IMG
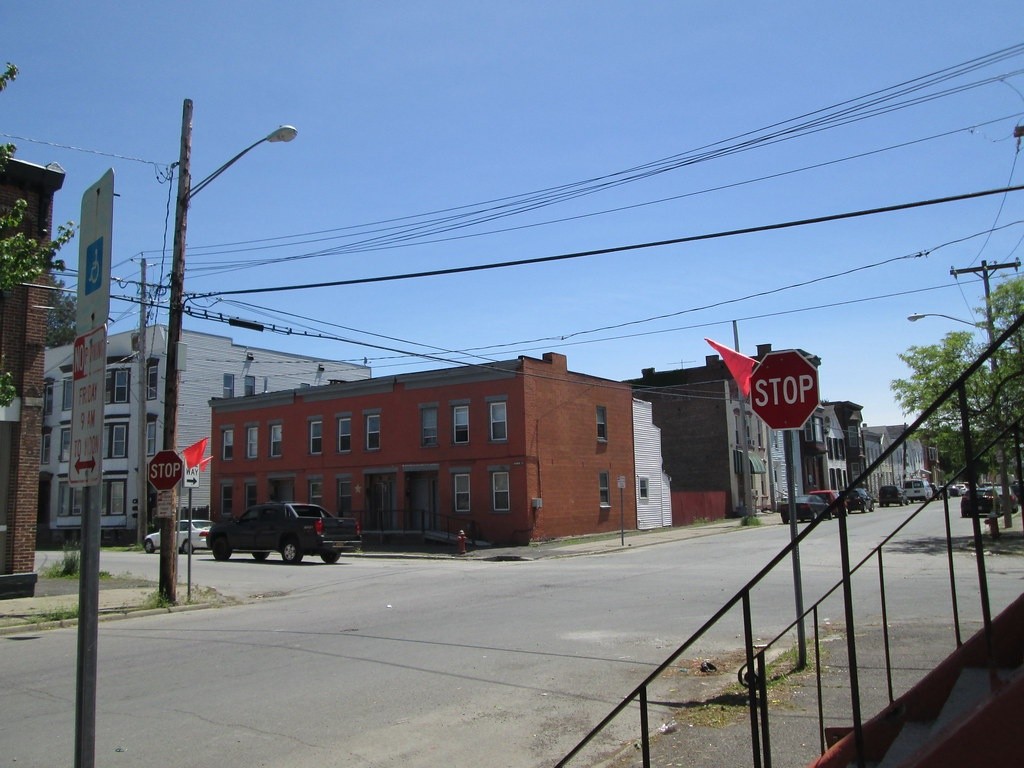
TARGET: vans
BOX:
[903,479,935,503]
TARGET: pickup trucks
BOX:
[204,501,362,566]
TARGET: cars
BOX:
[841,487,876,514]
[878,485,910,507]
[808,489,849,520]
[929,480,968,500]
[781,494,834,525]
[144,519,216,554]
[960,488,1001,519]
[984,486,1020,515]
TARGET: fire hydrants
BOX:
[458,528,469,554]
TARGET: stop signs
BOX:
[148,449,184,492]
[748,350,821,430]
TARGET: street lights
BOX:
[905,311,1015,530]
[157,120,301,608]
